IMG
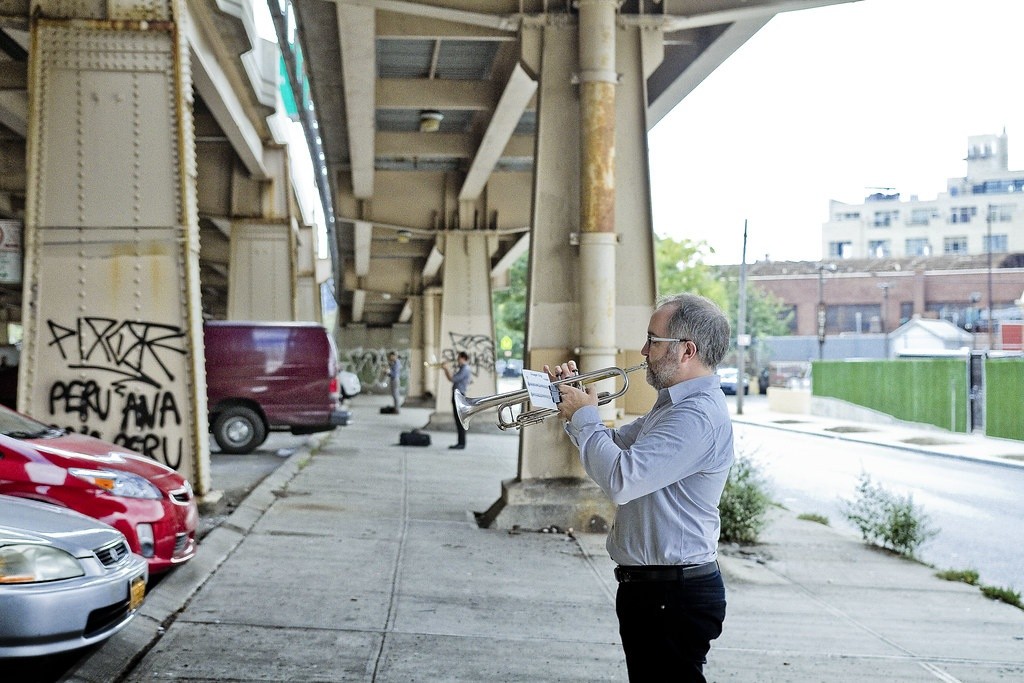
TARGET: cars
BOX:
[716,367,749,395]
[0,397,198,575]
[0,490,149,661]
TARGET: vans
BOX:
[758,361,807,395]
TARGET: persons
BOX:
[441,352,474,450]
[386,352,402,411]
[543,293,736,683]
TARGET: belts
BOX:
[614,560,719,582]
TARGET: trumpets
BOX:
[451,362,649,432]
[423,359,458,369]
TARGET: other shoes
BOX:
[449,444,464,449]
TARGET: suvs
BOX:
[1,321,361,454]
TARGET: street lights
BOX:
[816,263,838,360]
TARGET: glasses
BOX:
[647,335,692,348]
